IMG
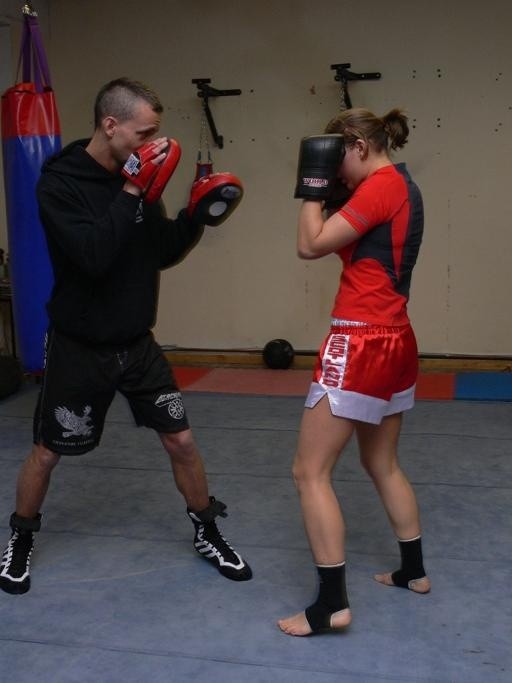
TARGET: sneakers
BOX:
[190,501,252,582]
[0,510,42,597]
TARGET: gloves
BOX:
[293,131,347,206]
[121,143,158,191]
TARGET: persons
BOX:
[269,104,436,641]
[1,73,255,597]
